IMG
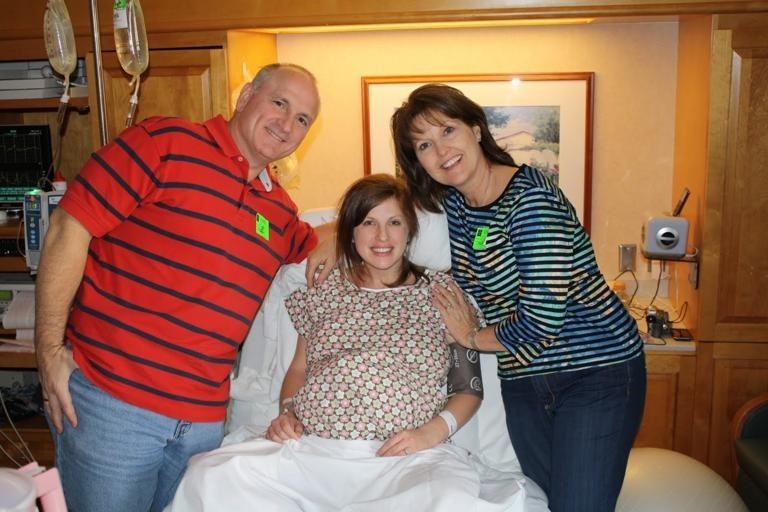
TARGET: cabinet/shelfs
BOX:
[0,30,276,469]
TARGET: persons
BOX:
[264,171,486,457]
[34,62,339,512]
[303,83,648,512]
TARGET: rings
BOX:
[43,398,48,401]
[447,302,454,309]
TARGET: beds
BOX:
[235,201,549,511]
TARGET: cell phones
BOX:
[672,327,695,341]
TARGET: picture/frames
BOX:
[359,71,593,240]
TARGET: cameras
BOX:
[645,305,668,338]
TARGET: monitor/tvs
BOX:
[0,123,54,216]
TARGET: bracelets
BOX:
[439,410,458,438]
[470,326,482,351]
